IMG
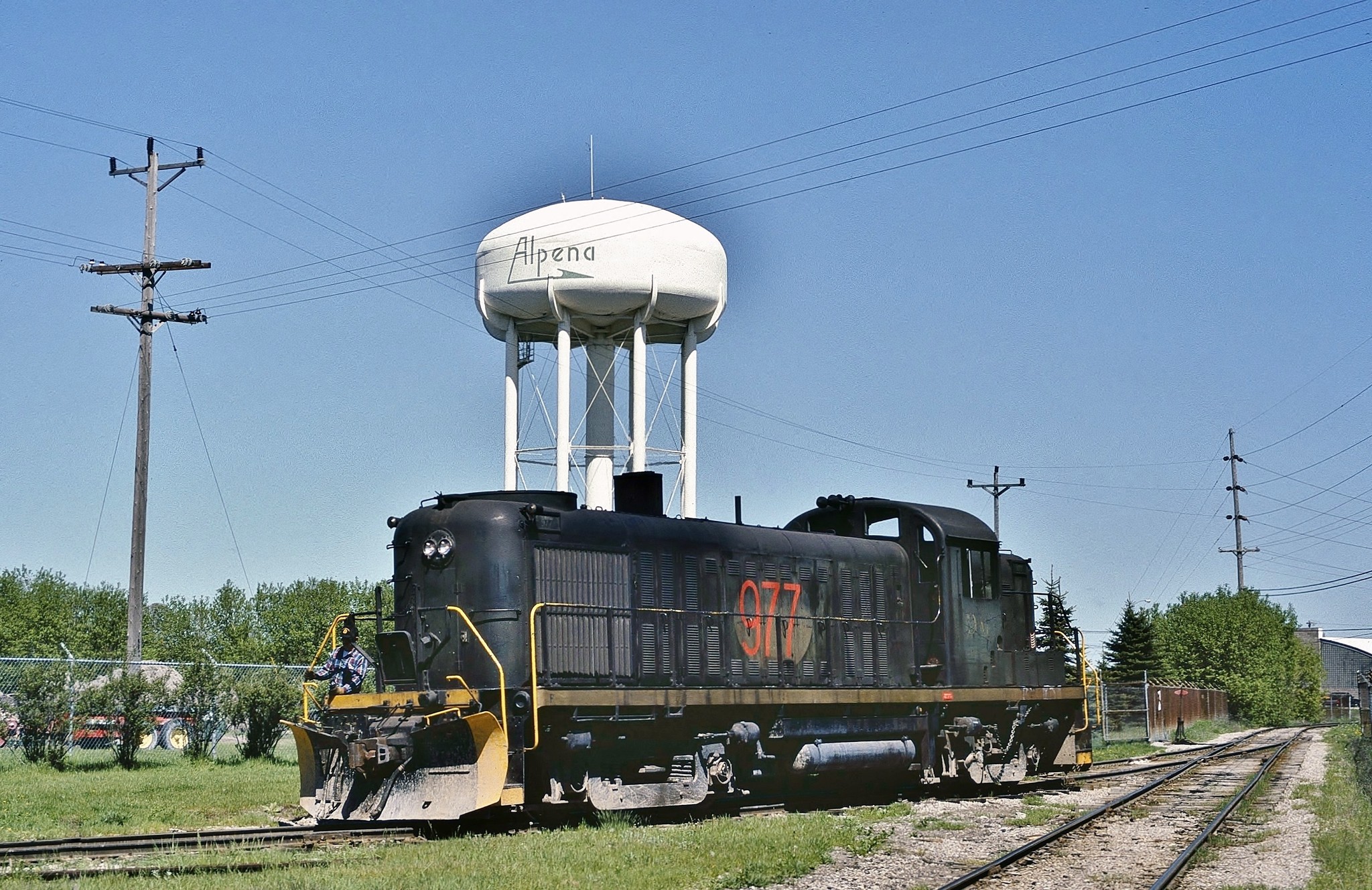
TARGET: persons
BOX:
[304,627,368,696]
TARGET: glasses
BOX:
[340,638,355,643]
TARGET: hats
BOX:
[337,625,359,638]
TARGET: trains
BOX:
[278,490,1102,833]
